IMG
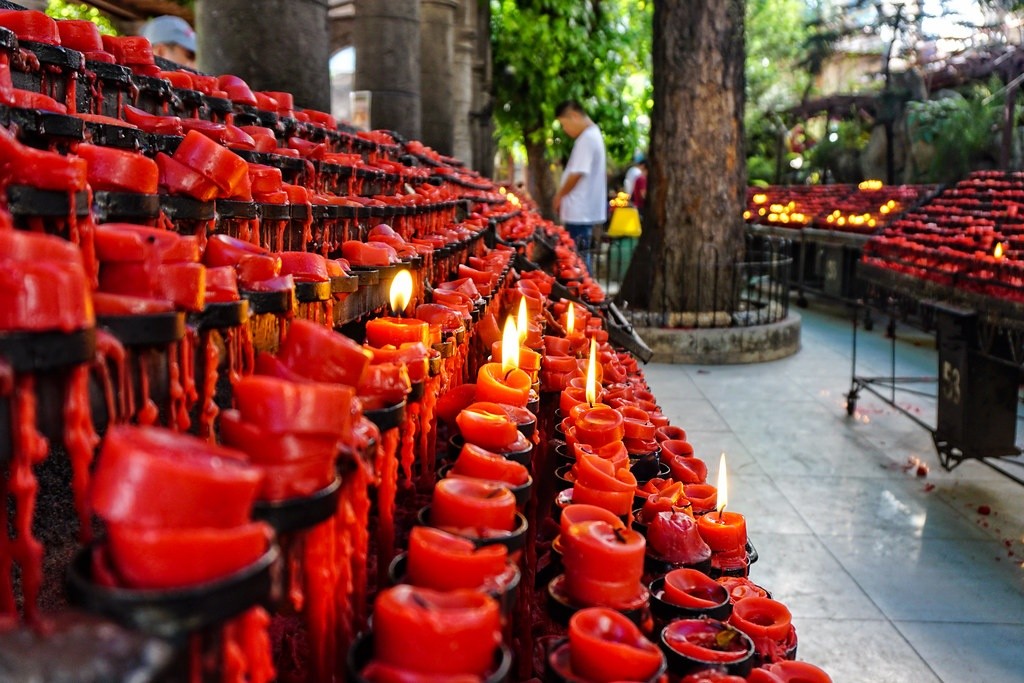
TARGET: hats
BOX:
[140,14,197,50]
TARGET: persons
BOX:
[551,99,648,274]
[141,15,200,70]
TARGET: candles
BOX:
[862,170,1024,303]
[0,9,832,683]
